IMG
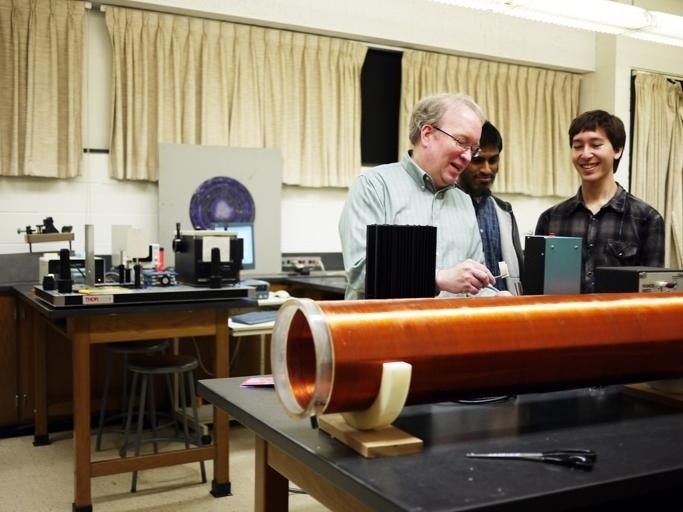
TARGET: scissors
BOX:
[465,448,597,470]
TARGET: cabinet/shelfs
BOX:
[0,249,97,442]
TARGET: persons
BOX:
[452,120,526,295]
[338,92,512,300]
[535,110,665,293]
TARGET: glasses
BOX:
[433,126,482,158]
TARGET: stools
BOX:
[95,334,181,449]
[119,351,210,493]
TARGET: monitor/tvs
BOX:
[210,222,255,270]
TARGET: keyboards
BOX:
[232,311,277,325]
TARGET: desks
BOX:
[10,279,256,512]
[283,275,351,302]
[190,356,683,509]
